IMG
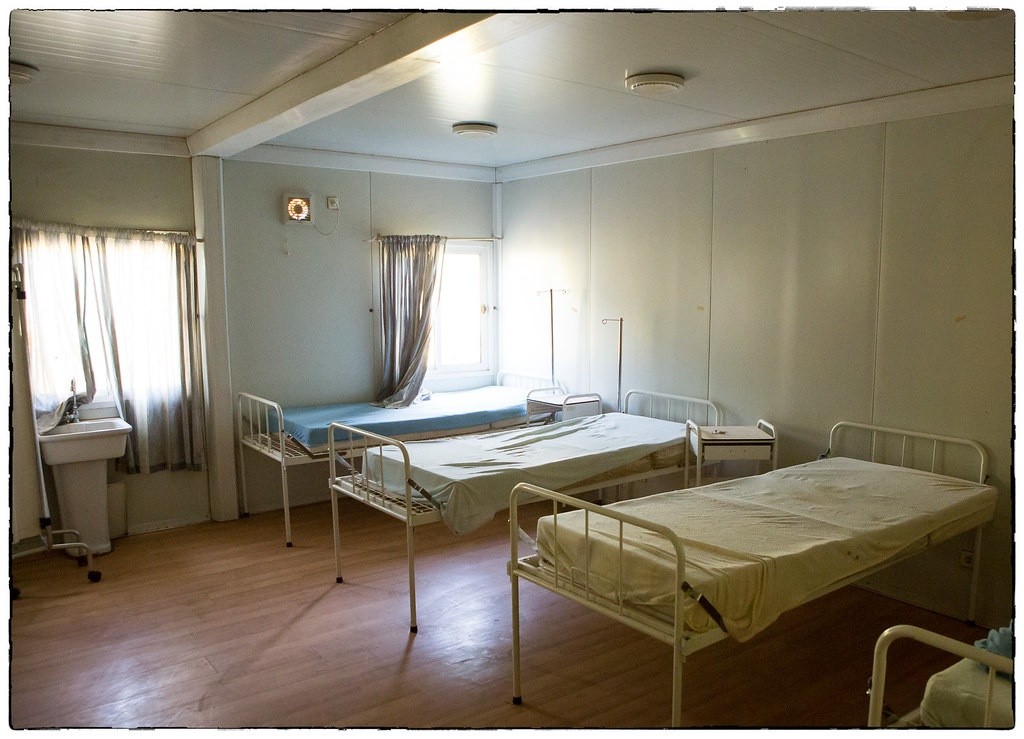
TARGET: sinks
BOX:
[39,418,132,464]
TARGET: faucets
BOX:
[64,379,80,423]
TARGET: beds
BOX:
[237,371,560,547]
[868,617,1014,727]
[507,421,998,727]
[328,390,719,633]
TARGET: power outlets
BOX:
[328,197,339,209]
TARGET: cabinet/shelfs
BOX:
[527,388,603,428]
[683,419,778,489]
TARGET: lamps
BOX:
[452,124,497,138]
[624,74,685,97]
[10,63,40,84]
